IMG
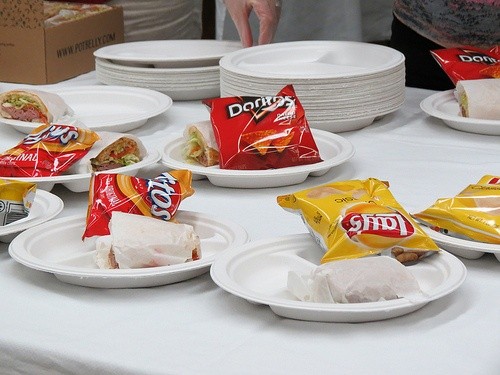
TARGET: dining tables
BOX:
[0,56,500,375]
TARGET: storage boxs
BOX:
[0,0,126,87]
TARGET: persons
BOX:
[81,0,282,49]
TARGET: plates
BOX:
[1,141,164,194]
[419,89,500,135]
[0,85,173,133]
[0,188,65,243]
[209,233,469,324]
[418,220,500,262]
[155,125,355,188]
[88,37,407,135]
[6,208,249,289]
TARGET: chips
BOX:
[0,44,500,266]
